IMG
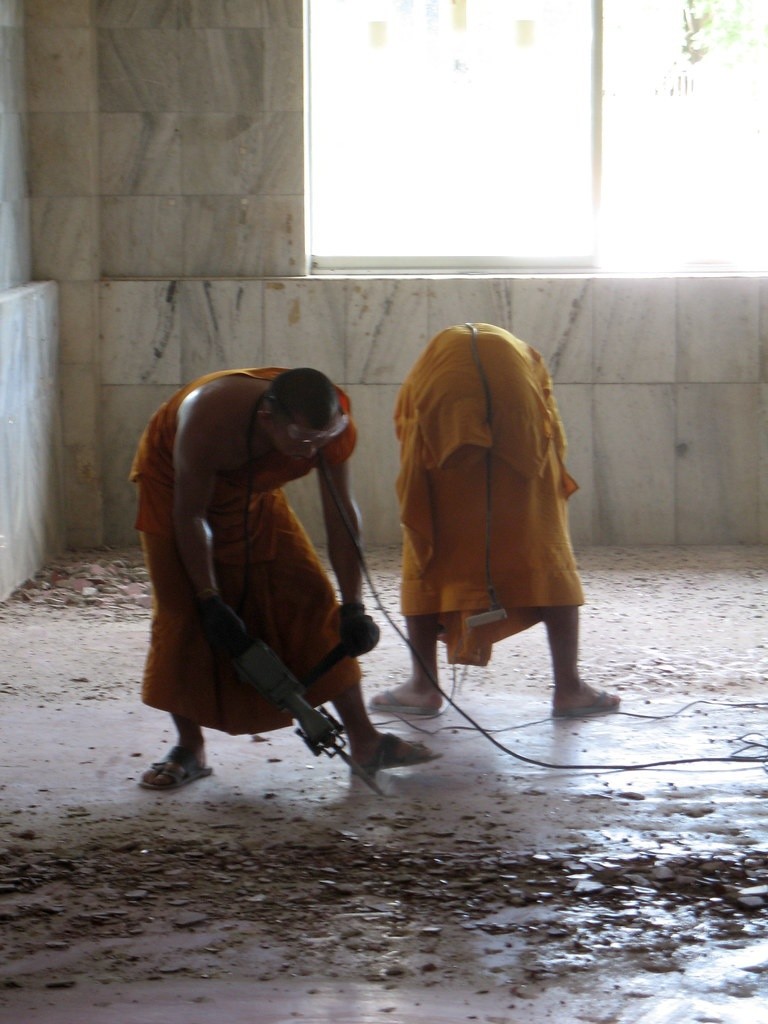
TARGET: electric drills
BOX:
[199,602,398,804]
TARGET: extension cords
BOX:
[466,607,507,628]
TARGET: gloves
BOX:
[200,595,245,658]
[340,603,380,659]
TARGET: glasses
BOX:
[273,396,347,445]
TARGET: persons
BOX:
[370,323,620,718]
[130,368,445,790]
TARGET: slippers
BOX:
[137,745,212,789]
[350,733,443,774]
[369,691,439,715]
[552,692,621,717]
